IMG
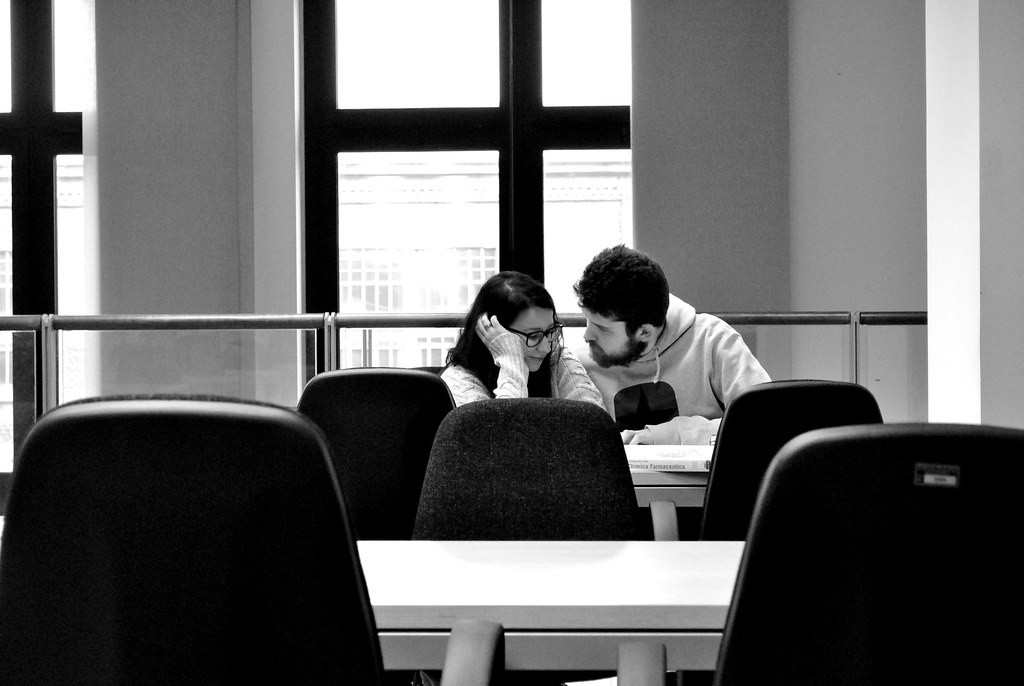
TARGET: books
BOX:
[615,440,716,473]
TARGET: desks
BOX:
[350,538,743,686]
[626,445,712,540]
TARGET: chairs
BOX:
[409,397,644,686]
[706,422,1024,686]
[676,380,886,686]
[1,396,385,686]
[297,367,455,540]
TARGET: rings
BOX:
[483,324,493,331]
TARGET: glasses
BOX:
[508,321,564,347]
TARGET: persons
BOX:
[563,243,774,445]
[439,270,610,418]
